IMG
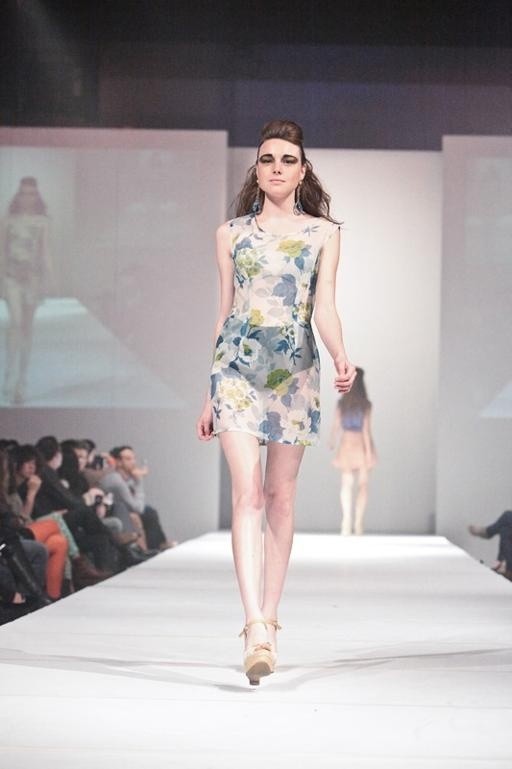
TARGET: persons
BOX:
[1,437,171,621]
[468,511,511,581]
[331,367,379,536]
[198,121,359,685]
[0,177,57,405]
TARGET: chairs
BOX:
[469,524,489,540]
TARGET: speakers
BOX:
[74,555,104,579]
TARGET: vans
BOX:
[239,620,282,685]
